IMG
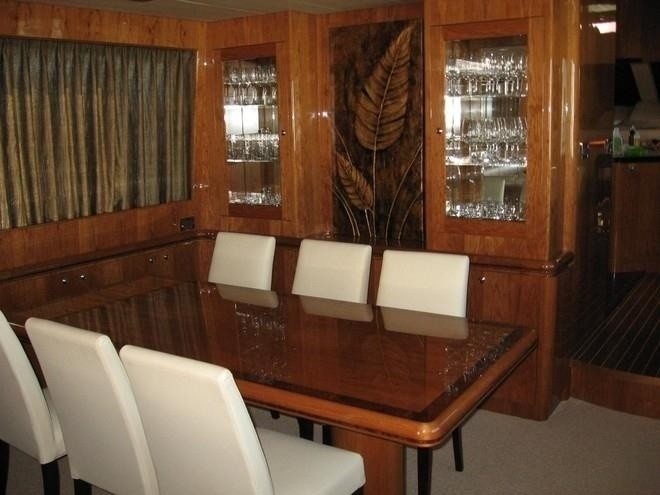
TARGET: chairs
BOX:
[1,311,67,495]
[119,345,366,495]
[373,249,471,495]
[25,317,161,495]
[289,239,373,444]
[207,231,280,421]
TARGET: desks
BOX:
[4,272,538,495]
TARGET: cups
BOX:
[229,184,282,209]
[229,133,280,160]
[445,197,522,221]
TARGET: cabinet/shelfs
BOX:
[610,157,660,278]
[205,10,317,239]
[421,0,565,264]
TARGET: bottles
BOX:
[628,126,640,146]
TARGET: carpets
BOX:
[573,272,659,380]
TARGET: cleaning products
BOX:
[612,121,625,157]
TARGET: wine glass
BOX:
[443,49,530,97]
[447,118,526,167]
[223,61,276,106]
